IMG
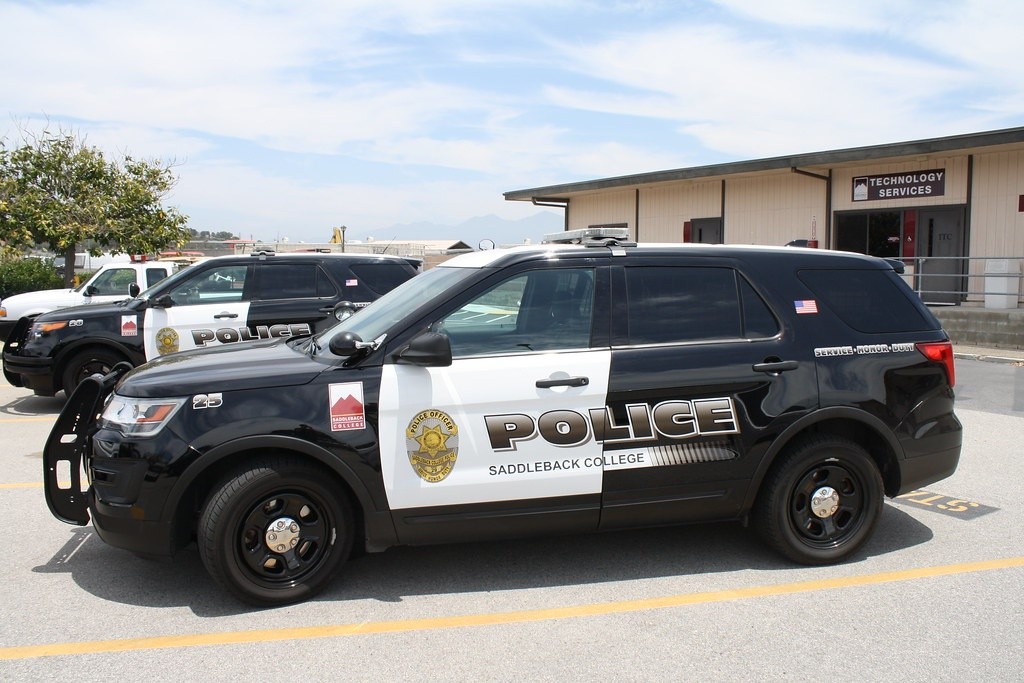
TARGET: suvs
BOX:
[39,226,964,604]
[0,250,426,401]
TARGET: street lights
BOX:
[340,226,347,253]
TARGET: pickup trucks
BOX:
[0,253,243,341]
